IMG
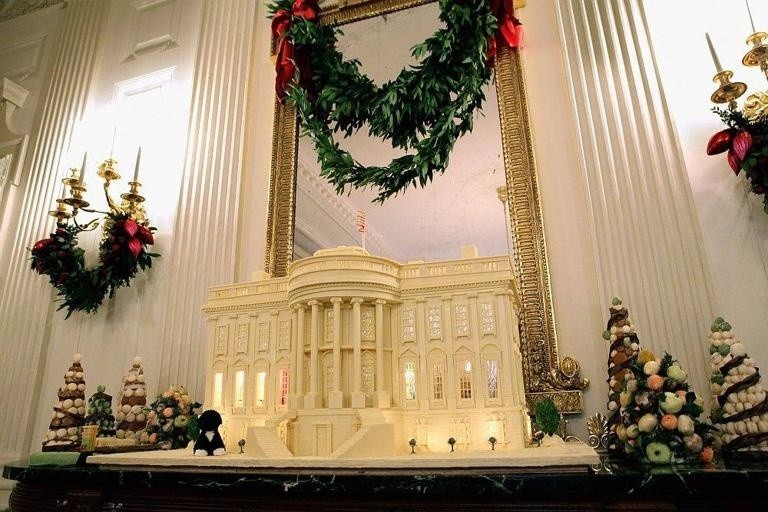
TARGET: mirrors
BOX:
[265,0,584,415]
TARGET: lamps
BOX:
[49,124,152,229]
[706,0,768,220]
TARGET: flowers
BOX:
[708,102,767,217]
[265,0,525,204]
[25,217,158,318]
